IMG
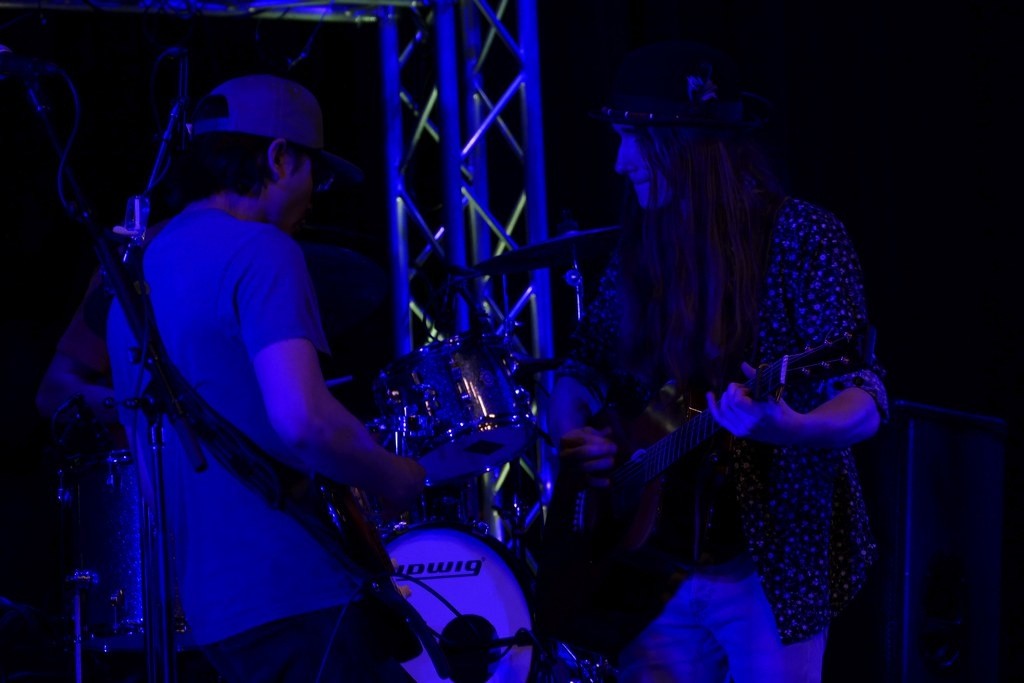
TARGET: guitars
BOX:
[538,323,877,579]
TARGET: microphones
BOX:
[0,43,53,115]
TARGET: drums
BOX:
[371,332,537,487]
[53,452,198,656]
[377,522,547,683]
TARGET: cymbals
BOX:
[473,223,623,274]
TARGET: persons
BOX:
[35,224,213,682]
[105,78,425,683]
[550,39,891,683]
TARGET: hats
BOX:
[185,75,365,185]
[587,39,773,128]
